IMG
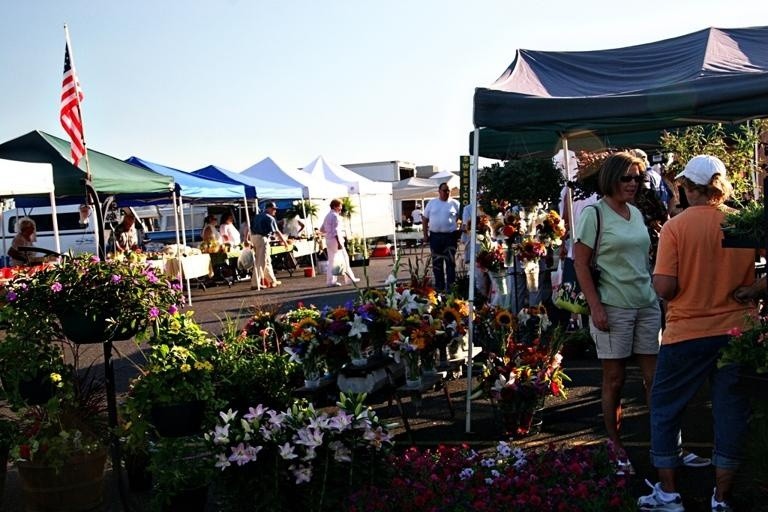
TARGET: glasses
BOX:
[621,175,644,183]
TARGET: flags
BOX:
[60,41,86,168]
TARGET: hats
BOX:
[674,154,726,187]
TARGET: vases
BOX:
[57,305,145,341]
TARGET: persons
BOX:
[557,150,768,512]
[199,202,304,291]
[320,199,361,287]
[423,183,461,294]
[410,204,423,226]
[108,214,141,255]
[461,199,483,244]
[9,218,37,266]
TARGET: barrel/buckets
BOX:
[318,260,328,275]
[305,268,312,277]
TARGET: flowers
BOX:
[16,339,662,509]
[46,256,178,306]
[431,200,578,342]
[256,284,430,334]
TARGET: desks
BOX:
[145,196,428,311]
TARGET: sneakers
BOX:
[250,285,266,290]
[711,487,730,512]
[328,282,342,287]
[608,456,636,476]
[271,281,281,288]
[636,478,685,512]
[345,278,360,285]
[676,448,712,467]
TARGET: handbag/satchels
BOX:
[555,205,600,315]
[551,258,563,310]
[199,240,220,254]
